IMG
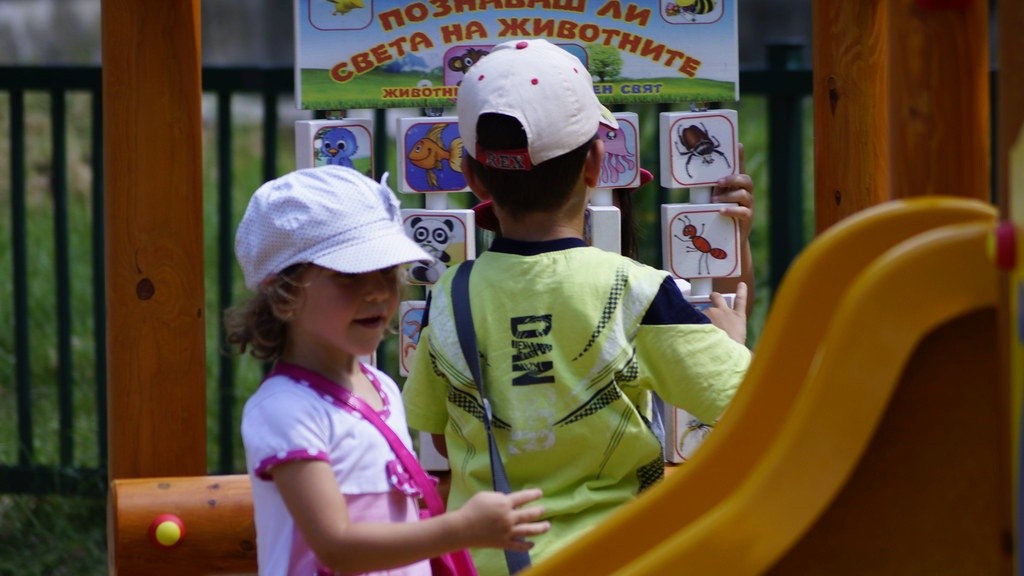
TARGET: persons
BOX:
[220,163,551,576]
[401,40,753,576]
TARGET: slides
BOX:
[499,195,1003,575]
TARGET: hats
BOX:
[232,163,435,296]
[456,39,619,171]
